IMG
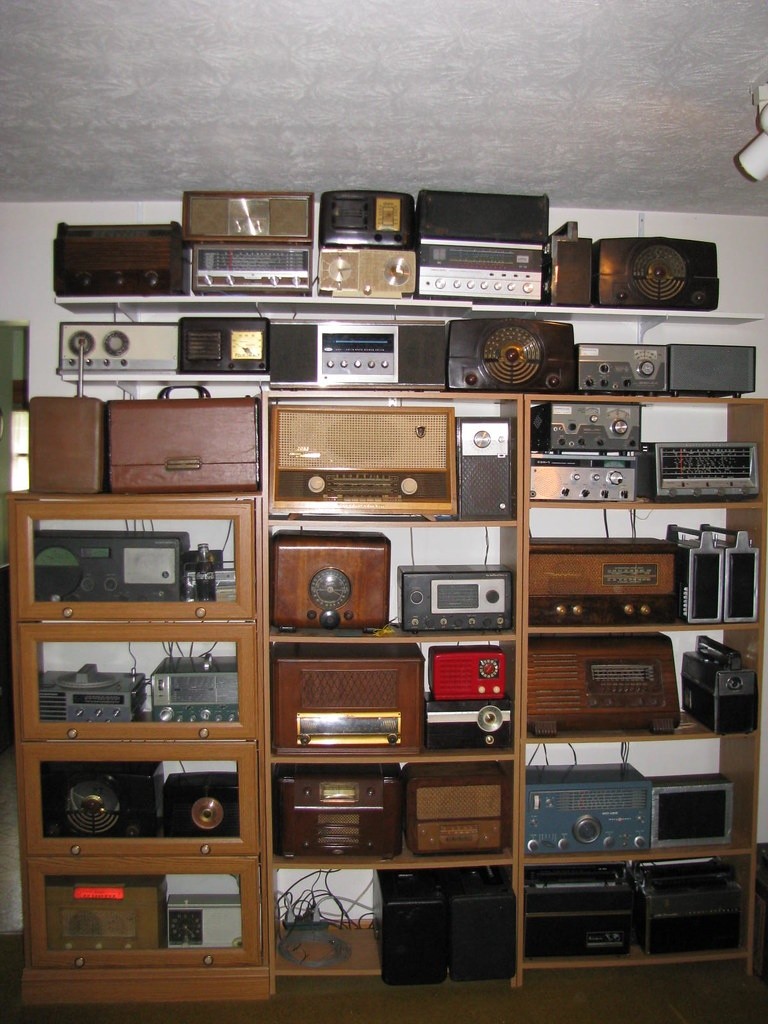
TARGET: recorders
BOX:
[33,189,763,985]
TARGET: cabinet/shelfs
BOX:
[1,487,271,1005]
[524,392,768,994]
[54,296,766,399]
[255,387,524,990]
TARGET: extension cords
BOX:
[282,919,329,931]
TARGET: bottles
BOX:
[196,544,216,601]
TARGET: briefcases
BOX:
[107,385,259,494]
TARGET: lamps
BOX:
[739,82,768,180]
